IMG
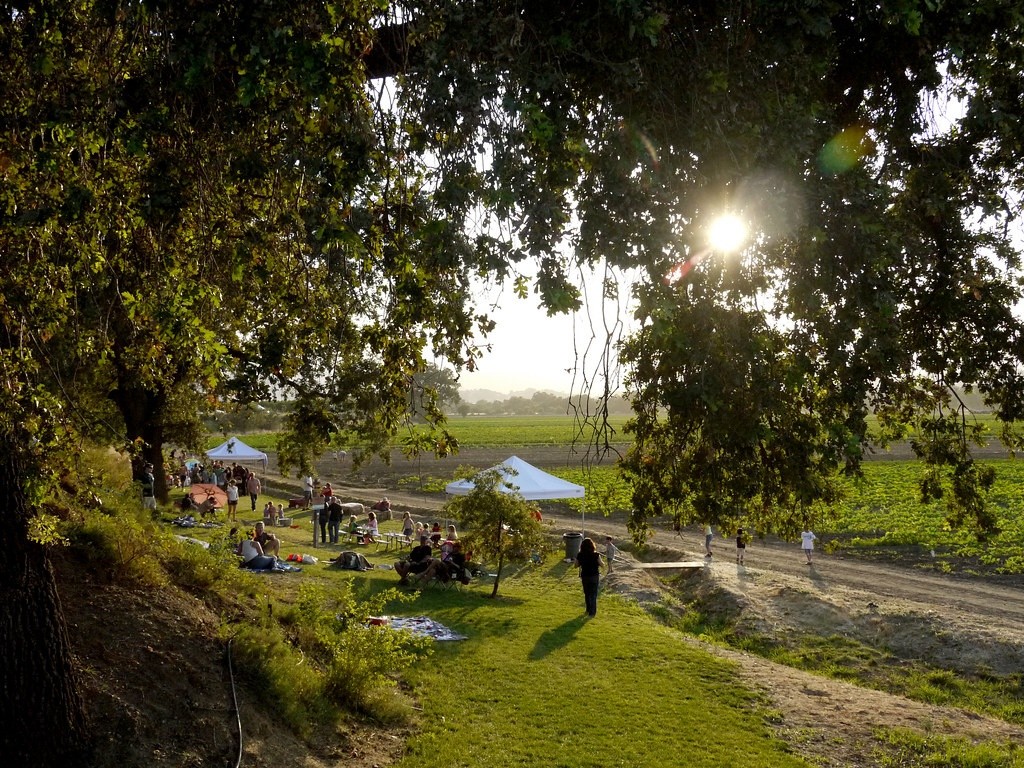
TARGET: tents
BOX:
[205,436,268,494]
[446,456,584,539]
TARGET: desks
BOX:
[343,523,376,543]
[384,532,406,551]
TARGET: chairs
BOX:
[409,570,465,593]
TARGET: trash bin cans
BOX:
[562,532,583,559]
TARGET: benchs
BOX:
[338,529,383,544]
[375,539,411,551]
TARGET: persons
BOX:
[168,448,250,497]
[605,538,621,574]
[673,523,683,540]
[225,528,239,555]
[255,474,466,592]
[800,525,816,565]
[237,529,284,573]
[574,537,605,616]
[704,525,713,558]
[140,463,156,509]
[340,449,347,462]
[333,450,337,461]
[246,472,261,512]
[60,469,77,494]
[226,479,239,522]
[198,499,217,519]
[189,493,200,506]
[180,493,198,512]
[735,529,745,565]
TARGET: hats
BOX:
[453,543,462,547]
